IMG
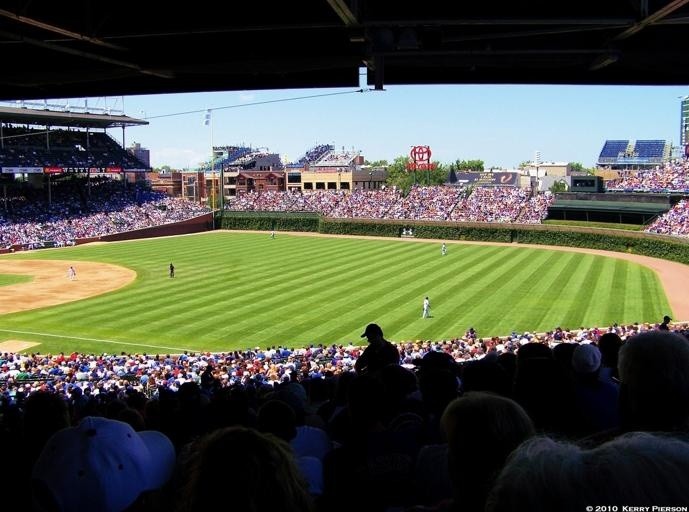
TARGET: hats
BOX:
[573,343,601,373]
[361,323,383,338]
[38,415,178,512]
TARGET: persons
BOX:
[2,315,688,511]
[441,243,447,255]
[604,154,689,237]
[169,263,175,278]
[68,267,76,281]
[422,296,431,318]
[3,123,556,249]
[0,124,143,168]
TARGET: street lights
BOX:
[204,108,216,230]
[535,150,543,202]
